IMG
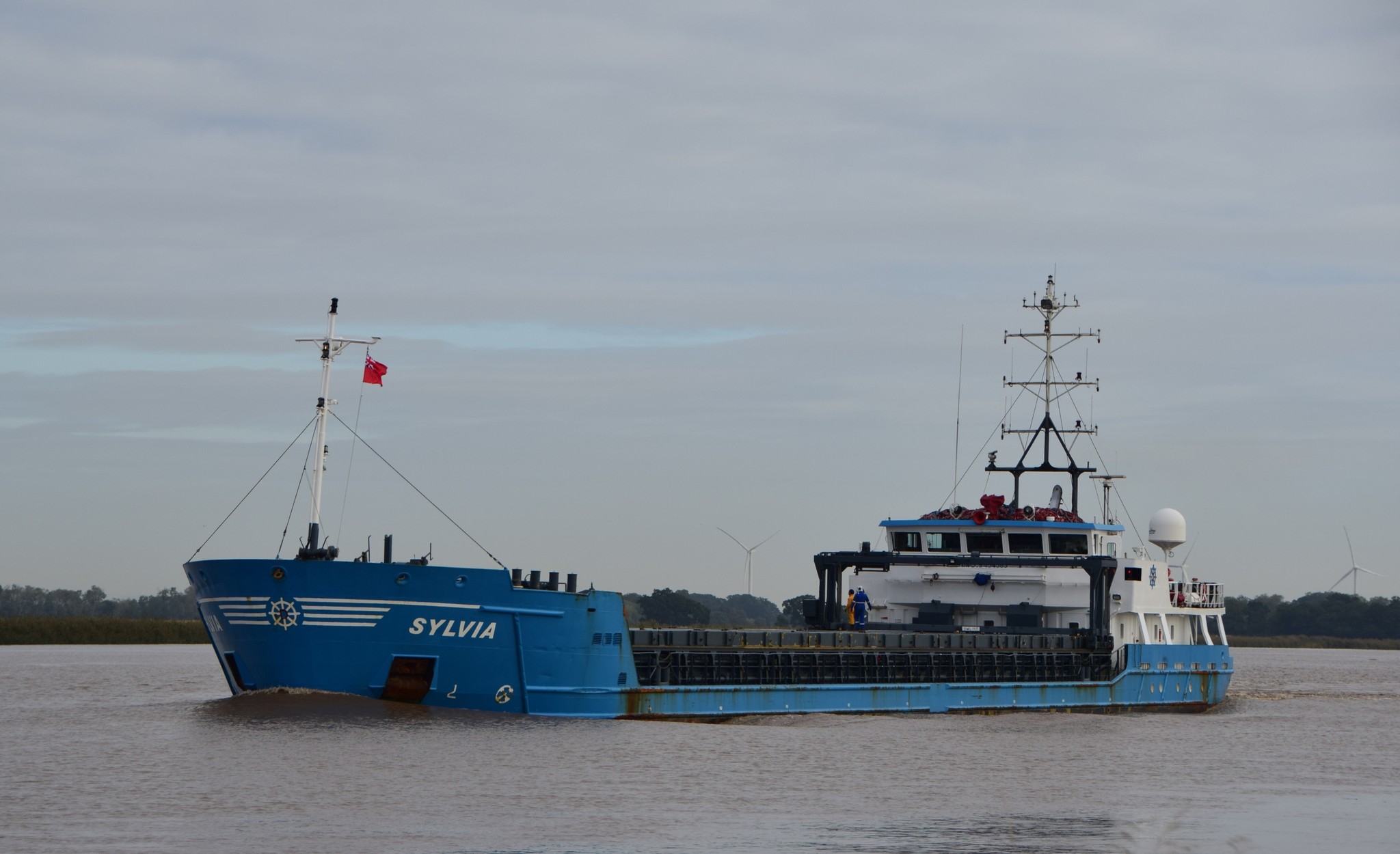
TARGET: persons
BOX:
[850,586,872,633]
[844,589,855,631]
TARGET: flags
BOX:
[363,354,387,387]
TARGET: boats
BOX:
[180,272,1236,720]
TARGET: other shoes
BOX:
[859,629,864,632]
[849,624,854,630]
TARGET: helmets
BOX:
[857,586,863,592]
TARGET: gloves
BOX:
[868,607,872,611]
[850,608,853,612]
